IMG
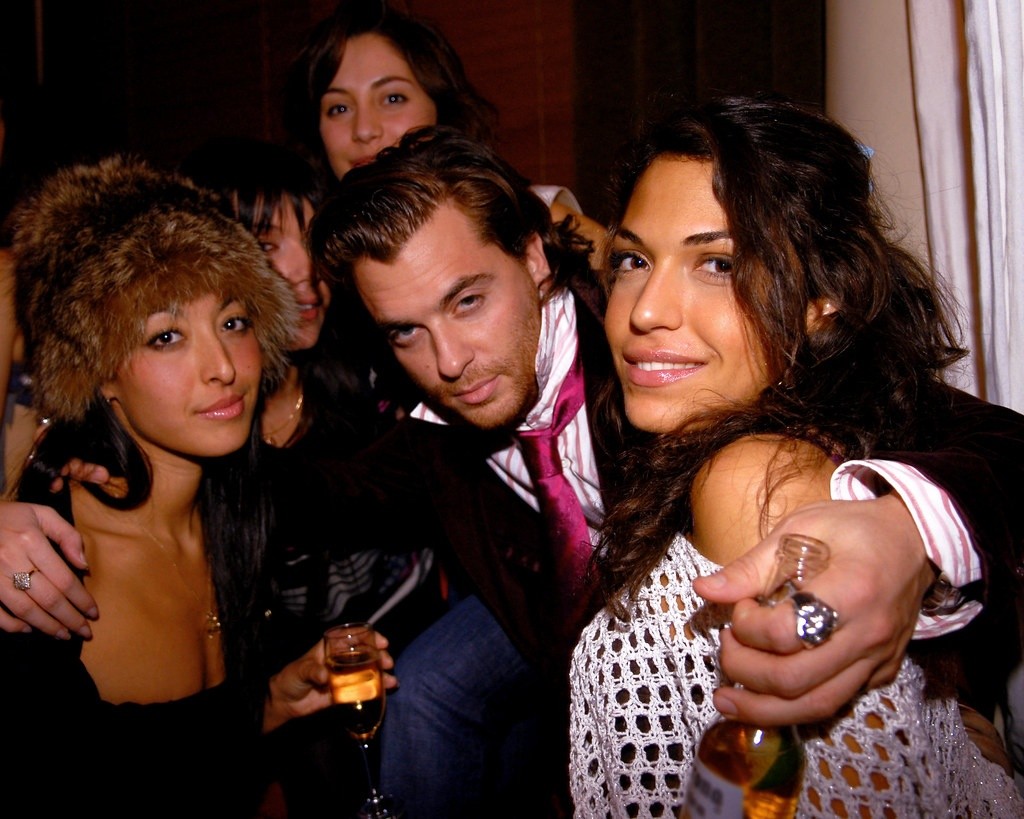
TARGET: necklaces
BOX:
[106,482,222,640]
[264,393,304,445]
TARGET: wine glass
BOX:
[322,622,398,819]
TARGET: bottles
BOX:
[677,534,830,819]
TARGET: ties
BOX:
[510,330,604,661]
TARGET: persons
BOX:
[0,1,1024,819]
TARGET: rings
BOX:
[12,568,39,592]
[790,591,838,651]
